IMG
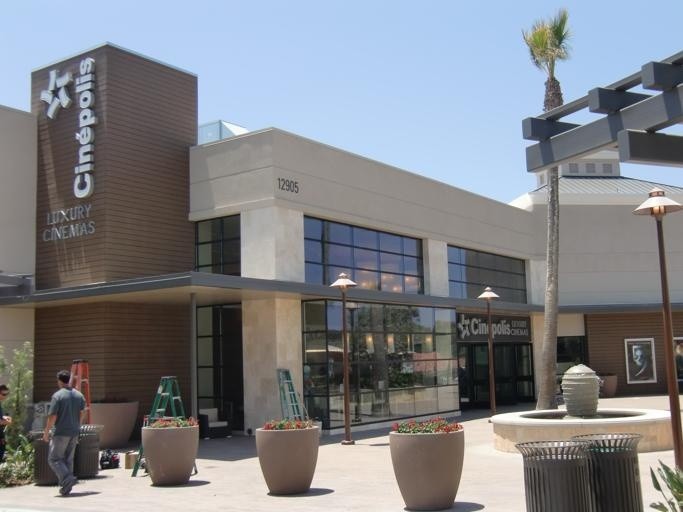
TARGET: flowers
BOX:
[144,416,198,428]
[259,416,314,430]
[393,416,464,433]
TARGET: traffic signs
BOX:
[631,186,682,476]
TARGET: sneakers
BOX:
[60,477,77,496]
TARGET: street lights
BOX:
[330,272,358,446]
[480,286,499,413]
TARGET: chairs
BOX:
[198,408,233,440]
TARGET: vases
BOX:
[256,426,319,494]
[389,432,465,510]
[87,402,139,447]
[142,425,200,486]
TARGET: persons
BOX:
[42,370,86,494]
[0,385,12,462]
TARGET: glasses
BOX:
[1,392,9,396]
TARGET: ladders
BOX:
[66,358,92,423]
[276,368,303,422]
[131,375,198,477]
